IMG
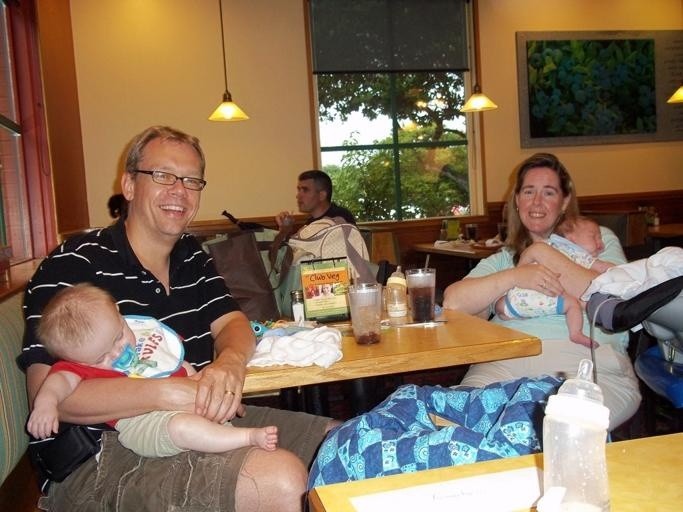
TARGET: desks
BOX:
[241,308,543,394]
[306,432,683,512]
[414,241,507,262]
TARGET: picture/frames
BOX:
[514,29,683,150]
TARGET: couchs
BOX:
[580,211,647,248]
[0,284,49,512]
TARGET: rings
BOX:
[540,278,548,287]
[224,390,234,396]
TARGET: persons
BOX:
[275,168,358,233]
[441,151,643,434]
[489,216,617,350]
[14,126,344,512]
[25,282,278,459]
[317,283,333,298]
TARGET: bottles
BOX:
[386,265,407,318]
[542,357,613,511]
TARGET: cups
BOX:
[406,268,436,324]
[465,223,478,242]
[347,282,383,345]
[292,290,304,322]
[498,223,507,243]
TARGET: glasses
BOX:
[131,168,206,190]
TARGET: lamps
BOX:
[207,0,251,123]
[666,84,683,105]
[458,1,498,114]
